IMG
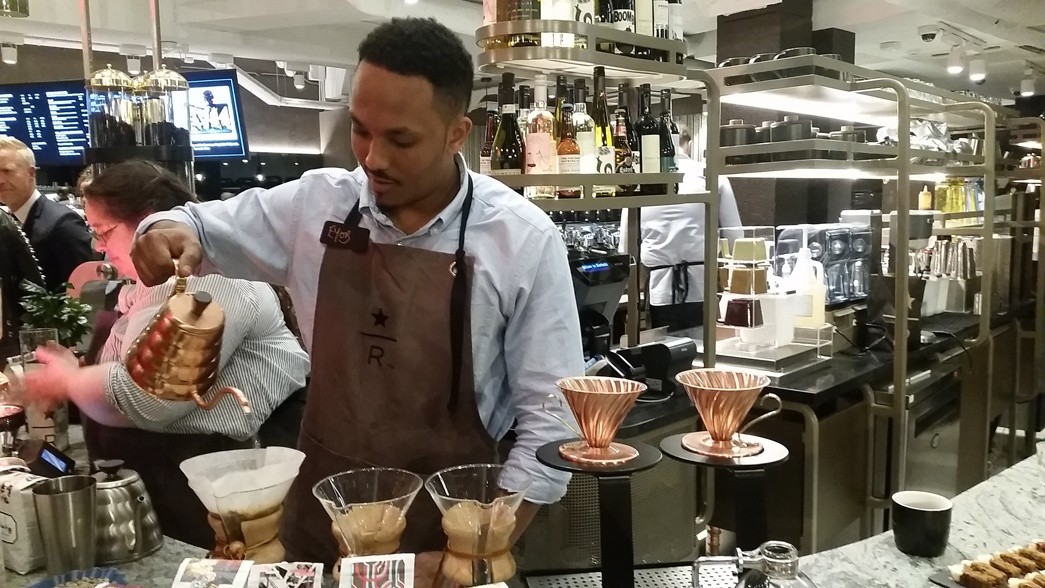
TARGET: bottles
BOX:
[471,1,684,198]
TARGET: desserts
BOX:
[948,539,1045,588]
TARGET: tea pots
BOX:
[124,259,252,415]
[86,458,163,564]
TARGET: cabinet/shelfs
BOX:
[472,15,1045,573]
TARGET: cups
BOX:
[35,475,96,576]
[674,367,783,456]
[892,491,953,556]
[21,332,73,452]
[920,176,982,279]
[545,377,648,465]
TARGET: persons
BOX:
[0,137,97,291]
[24,161,308,549]
[78,162,104,210]
[620,119,745,330]
[124,18,586,586]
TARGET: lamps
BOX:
[2,30,326,95]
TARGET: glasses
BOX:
[86,222,120,244]
